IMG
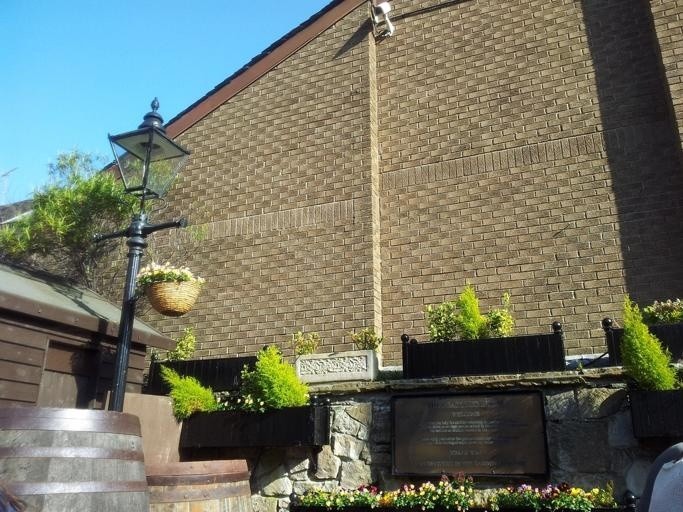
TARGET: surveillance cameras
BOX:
[374,1,391,17]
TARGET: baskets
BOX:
[144,279,201,318]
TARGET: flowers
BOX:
[129,253,205,285]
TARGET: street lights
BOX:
[90,94,191,412]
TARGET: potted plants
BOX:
[287,323,385,386]
[160,345,331,456]
[613,291,683,447]
[398,281,568,375]
[596,294,682,365]
[144,329,283,395]
